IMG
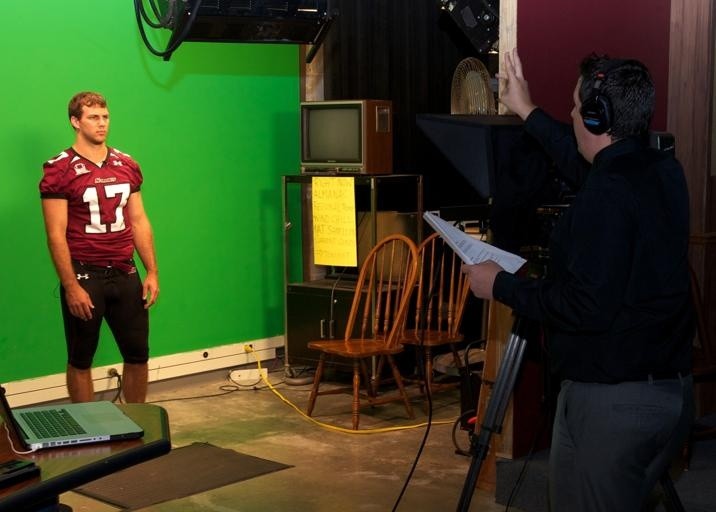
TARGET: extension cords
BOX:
[230,368,268,382]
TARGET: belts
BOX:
[81,259,137,274]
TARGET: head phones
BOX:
[582,59,648,135]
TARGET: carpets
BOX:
[70,442,295,511]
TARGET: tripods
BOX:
[457,267,686,512]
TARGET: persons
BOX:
[459,43,694,511]
[37,89,160,405]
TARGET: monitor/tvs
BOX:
[302,98,393,175]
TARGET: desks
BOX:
[0,403,171,512]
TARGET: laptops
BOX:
[0,388,145,452]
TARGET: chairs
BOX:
[305,234,418,431]
[396,225,491,416]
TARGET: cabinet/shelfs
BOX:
[281,174,424,374]
